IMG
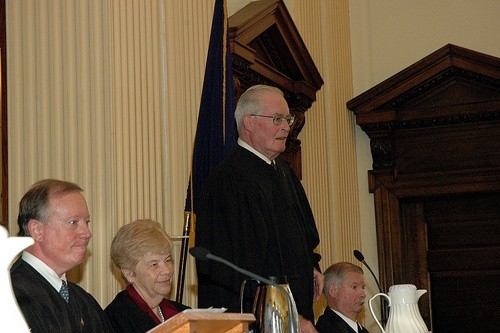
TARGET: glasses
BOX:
[250,114,295,126]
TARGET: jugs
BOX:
[0,224,35,333]
[368,284,429,333]
[238,275,303,333]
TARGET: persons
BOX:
[316,262,370,333]
[104,218,192,333]
[195,85,325,333]
[9,178,104,333]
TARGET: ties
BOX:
[356,322,362,333]
[59,280,69,304]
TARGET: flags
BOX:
[183,0,237,213]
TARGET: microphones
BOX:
[189,246,297,333]
[354,250,385,294]
